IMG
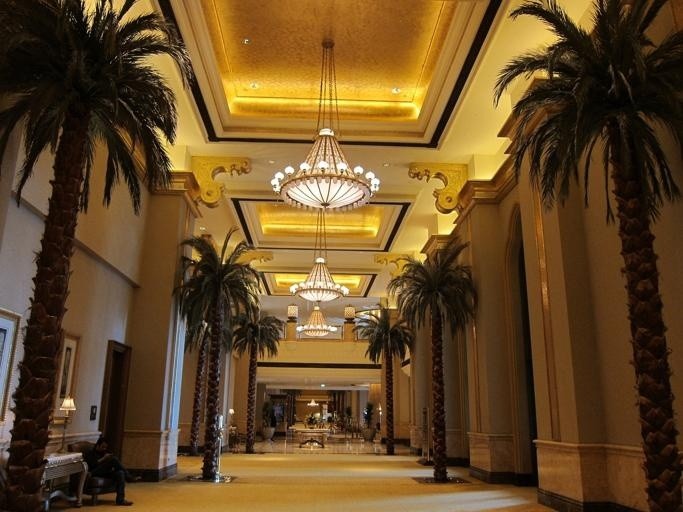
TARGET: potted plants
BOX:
[362,401,376,443]
[260,399,277,443]
[303,412,318,428]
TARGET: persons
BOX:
[80,437,141,506]
[270,401,284,427]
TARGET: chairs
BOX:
[64,440,124,506]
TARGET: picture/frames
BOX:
[1,308,84,429]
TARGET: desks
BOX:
[1,450,89,512]
[294,428,331,448]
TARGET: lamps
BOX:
[228,408,235,427]
[57,393,77,454]
[307,398,319,408]
[269,34,382,340]
